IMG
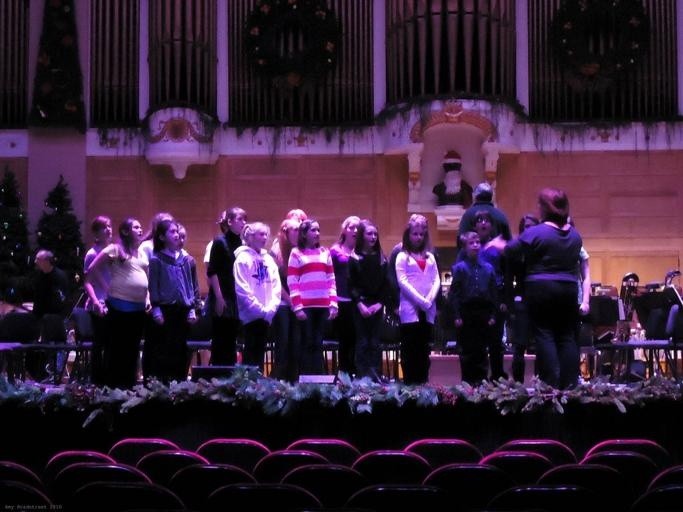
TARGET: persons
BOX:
[349,219,388,378]
[81,215,117,385]
[395,222,441,384]
[148,219,196,380]
[279,218,302,287]
[205,206,246,362]
[330,215,362,374]
[459,182,513,243]
[30,248,71,348]
[175,224,202,376]
[286,218,339,374]
[457,208,509,383]
[504,187,582,391]
[432,151,472,205]
[511,214,543,387]
[232,222,282,372]
[385,214,438,315]
[137,212,174,274]
[448,230,505,386]
[269,208,309,266]
[202,209,229,275]
[566,215,592,341]
[88,215,152,386]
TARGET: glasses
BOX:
[477,216,490,222]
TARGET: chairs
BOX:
[642,304,681,382]
